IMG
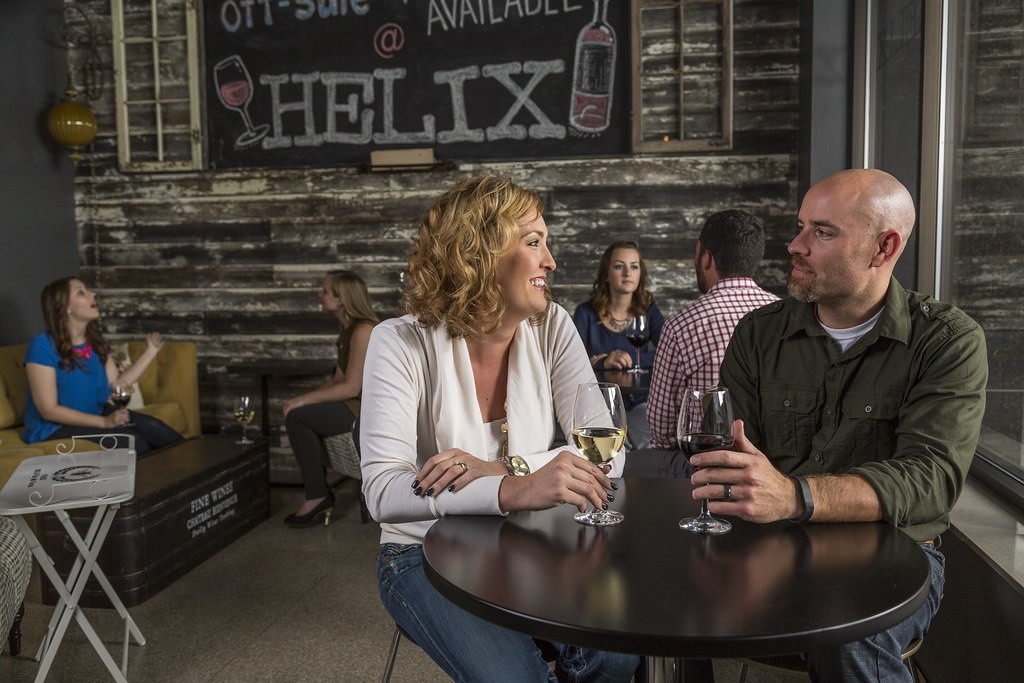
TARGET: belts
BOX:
[917,540,934,544]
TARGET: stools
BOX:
[1,433,146,683]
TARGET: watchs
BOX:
[499,455,529,477]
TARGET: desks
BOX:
[422,478,932,683]
[38,434,270,609]
[595,367,653,393]
[229,359,336,447]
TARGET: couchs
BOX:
[1,339,202,603]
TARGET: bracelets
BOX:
[789,476,814,524]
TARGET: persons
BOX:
[359,172,641,683]
[20,277,184,456]
[283,270,380,527]
[629,210,782,449]
[691,169,988,683]
[574,241,665,369]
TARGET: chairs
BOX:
[0,515,34,657]
[320,417,368,524]
[735,536,942,683]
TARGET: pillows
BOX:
[108,342,145,411]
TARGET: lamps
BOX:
[40,4,106,170]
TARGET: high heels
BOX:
[284,490,334,527]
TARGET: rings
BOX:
[724,483,731,502]
[458,463,467,473]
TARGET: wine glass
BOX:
[572,383,627,528]
[628,314,650,373]
[109,380,135,429]
[677,386,734,534]
[233,395,255,446]
